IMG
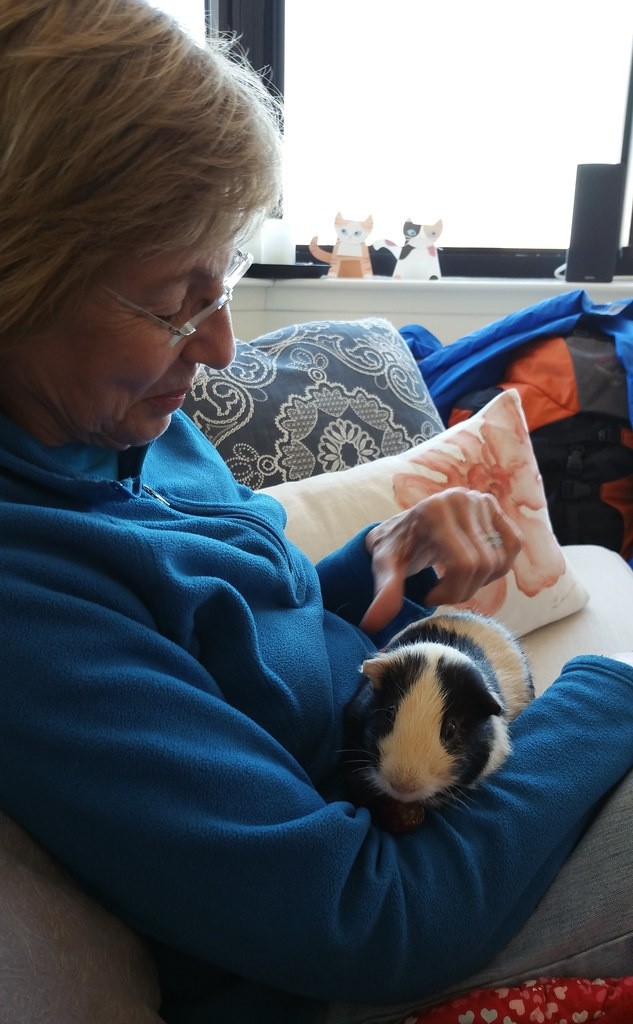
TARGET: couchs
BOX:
[0,543,633,1024]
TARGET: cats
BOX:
[373,215,443,280]
[308,210,374,280]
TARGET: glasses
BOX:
[92,246,254,348]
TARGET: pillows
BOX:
[176,317,447,492]
[253,384,589,640]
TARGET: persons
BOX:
[2,1,630,1023]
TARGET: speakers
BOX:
[566,163,626,282]
